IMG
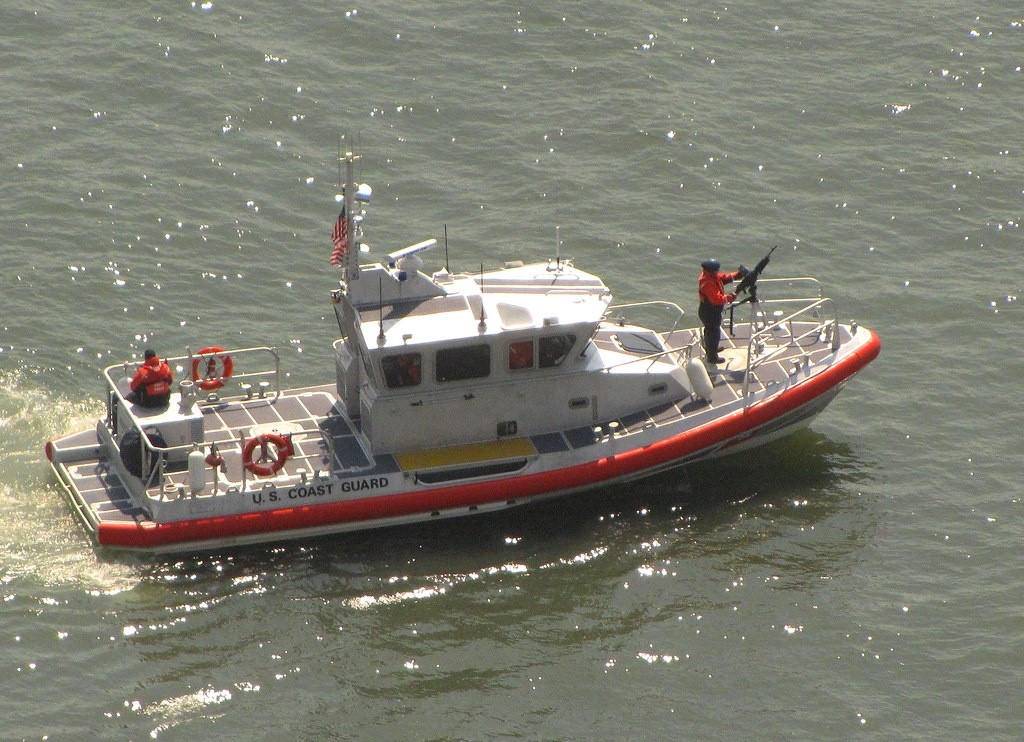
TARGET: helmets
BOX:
[701,259,721,271]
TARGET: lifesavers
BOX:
[189,345,236,389]
[242,432,290,478]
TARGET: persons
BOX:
[124,348,173,407]
[697,258,749,364]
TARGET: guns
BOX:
[729,241,776,309]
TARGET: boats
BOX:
[45,130,882,556]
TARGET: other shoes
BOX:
[708,357,725,363]
[717,345,724,351]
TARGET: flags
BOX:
[329,205,348,270]
